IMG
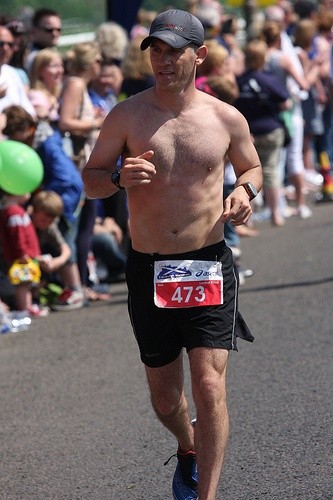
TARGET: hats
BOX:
[98,21,129,60]
[140,9,204,51]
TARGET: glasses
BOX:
[0,42,15,49]
[43,27,62,33]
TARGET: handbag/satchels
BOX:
[45,88,89,155]
[9,258,41,285]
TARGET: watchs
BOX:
[111,170,124,190]
[236,182,257,202]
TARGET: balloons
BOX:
[0,139,43,195]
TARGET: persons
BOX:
[80,10,263,500]
[0,8,155,333]
[190,0,333,256]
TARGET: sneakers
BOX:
[171,418,200,500]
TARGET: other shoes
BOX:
[282,208,299,217]
[297,207,311,219]
[17,306,50,319]
[50,290,83,311]
[84,287,110,301]
